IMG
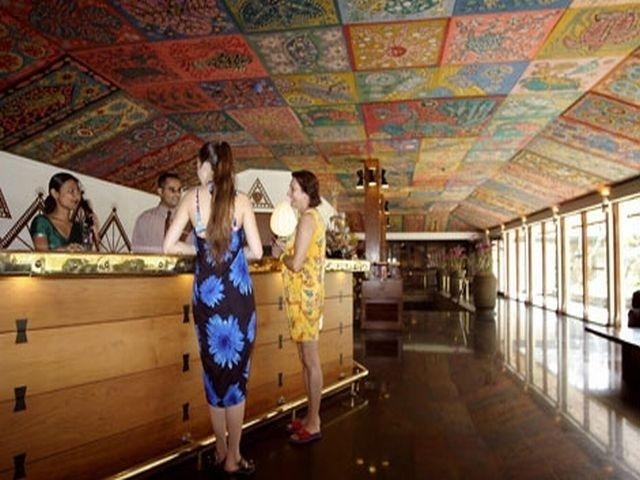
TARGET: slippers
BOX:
[285,421,303,434]
[211,451,253,467]
[223,458,256,475]
[288,427,322,444]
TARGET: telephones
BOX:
[80,197,93,226]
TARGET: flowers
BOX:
[426,241,499,273]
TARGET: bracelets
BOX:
[278,251,287,264]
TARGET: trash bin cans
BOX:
[426,267,438,287]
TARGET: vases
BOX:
[436,269,498,310]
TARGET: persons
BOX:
[130,171,195,259]
[269,169,328,445]
[160,138,264,478]
[31,172,92,256]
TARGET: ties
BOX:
[164,210,171,238]
